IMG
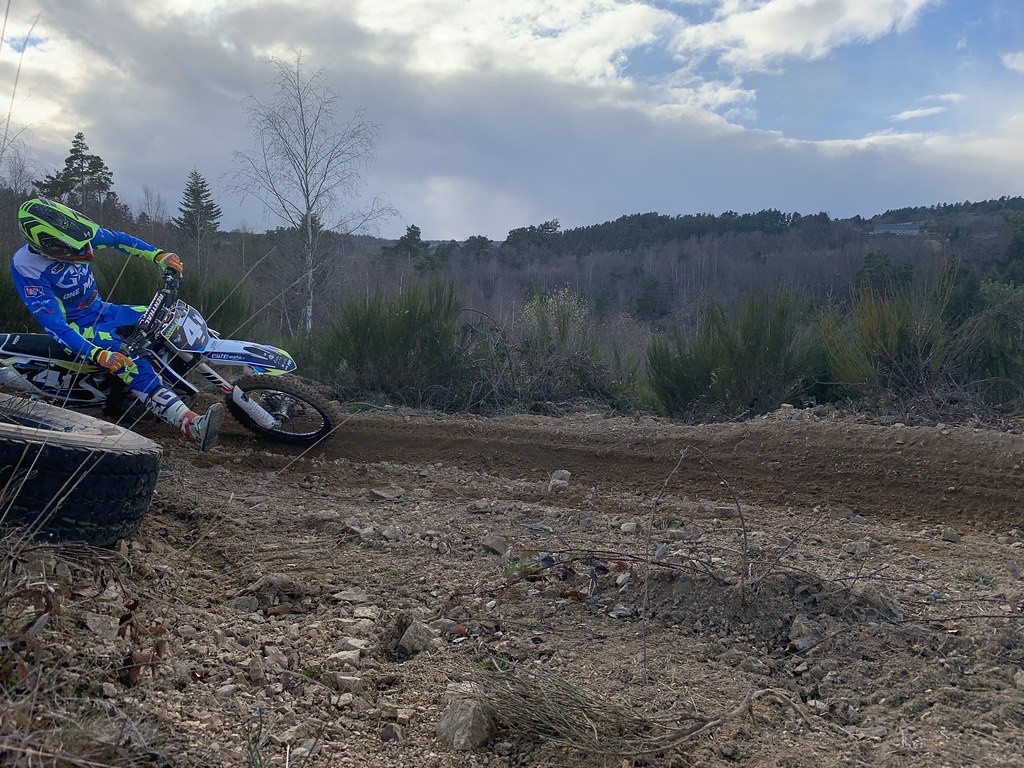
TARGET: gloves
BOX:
[155,251,184,273]
[94,348,133,375]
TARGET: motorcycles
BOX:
[0,261,340,449]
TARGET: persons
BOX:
[10,197,226,452]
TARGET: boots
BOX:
[144,384,225,452]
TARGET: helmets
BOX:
[18,197,100,261]
